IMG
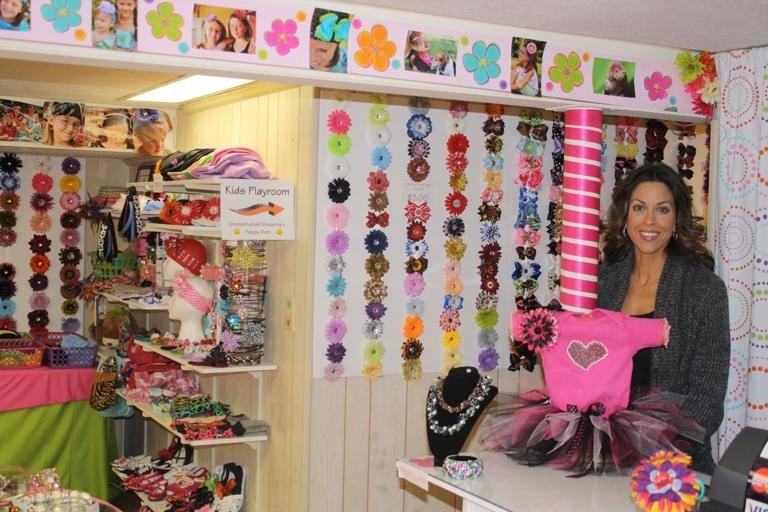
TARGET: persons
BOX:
[222,13,255,53]
[40,101,82,147]
[92,1,118,50]
[133,108,173,156]
[111,0,137,52]
[195,19,235,52]
[309,8,351,73]
[602,71,631,97]
[597,162,730,475]
[90,109,134,149]
[0,105,43,141]
[0,0,29,31]
[511,40,538,96]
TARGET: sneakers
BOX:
[110,435,247,512]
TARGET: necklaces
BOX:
[426,375,493,435]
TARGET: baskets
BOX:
[88,251,137,281]
[0,332,97,368]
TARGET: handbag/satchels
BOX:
[89,358,134,417]
[103,305,137,339]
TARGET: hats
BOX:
[88,186,128,209]
[165,237,207,276]
[113,193,162,216]
[148,199,220,227]
[51,102,81,119]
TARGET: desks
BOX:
[396,446,767,512]
[2,358,107,500]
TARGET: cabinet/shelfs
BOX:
[110,179,277,512]
